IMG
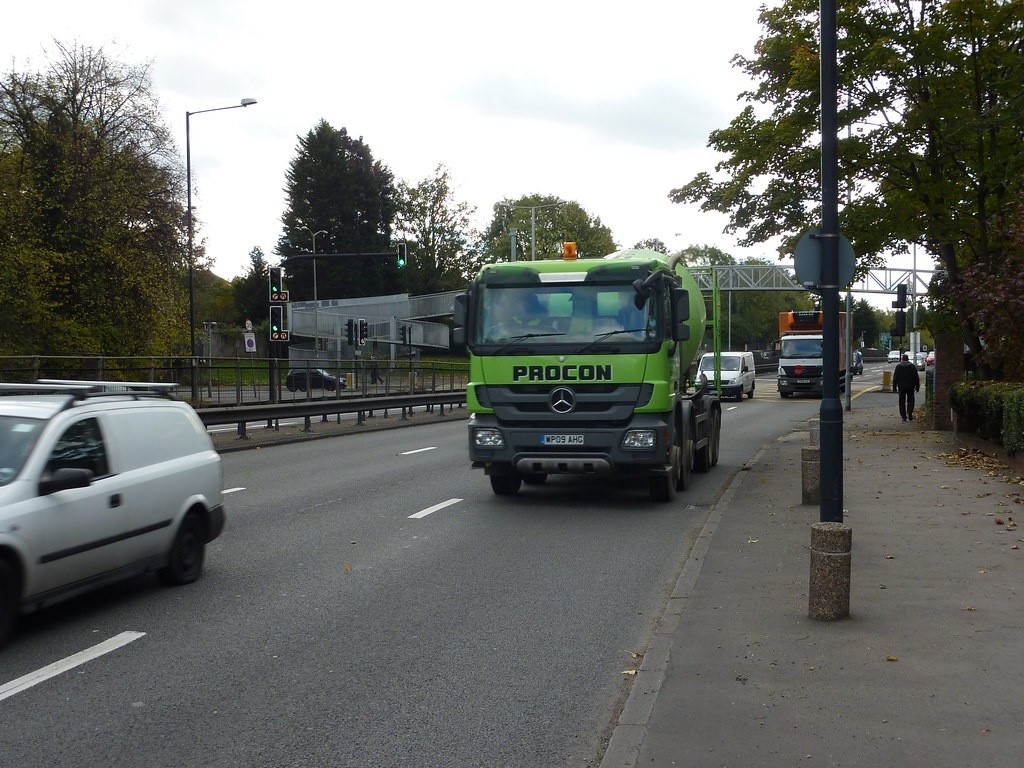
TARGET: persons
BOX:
[510,294,544,326]
[893,354,919,422]
[371,355,384,384]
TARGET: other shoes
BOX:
[910,418,914,420]
[901,419,906,422]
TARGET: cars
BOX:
[286,368,347,392]
[852,349,864,375]
[887,350,935,371]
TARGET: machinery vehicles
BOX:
[451,249,722,504]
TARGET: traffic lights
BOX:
[360,320,368,339]
[270,306,289,341]
[269,267,289,302]
[398,326,407,346]
[344,319,354,345]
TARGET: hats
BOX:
[902,354,908,361]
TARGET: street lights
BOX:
[301,225,328,370]
[861,330,867,349]
[185,97,258,399]
[500,198,567,262]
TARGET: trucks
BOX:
[777,311,854,399]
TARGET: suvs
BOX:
[0,379,228,635]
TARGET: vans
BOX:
[694,351,756,402]
